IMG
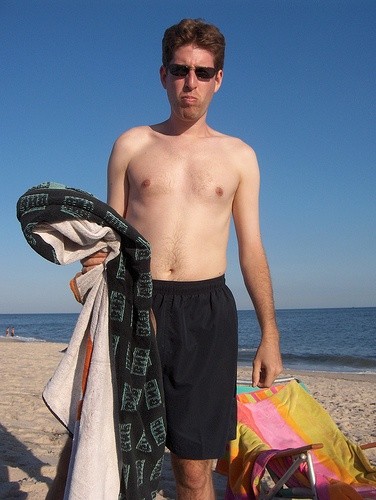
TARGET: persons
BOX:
[11,327,14,337]
[6,327,9,336]
[81,19,282,500]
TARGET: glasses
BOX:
[167,64,218,81]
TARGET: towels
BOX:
[215,379,376,500]
[16,182,167,500]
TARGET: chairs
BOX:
[215,377,376,500]
